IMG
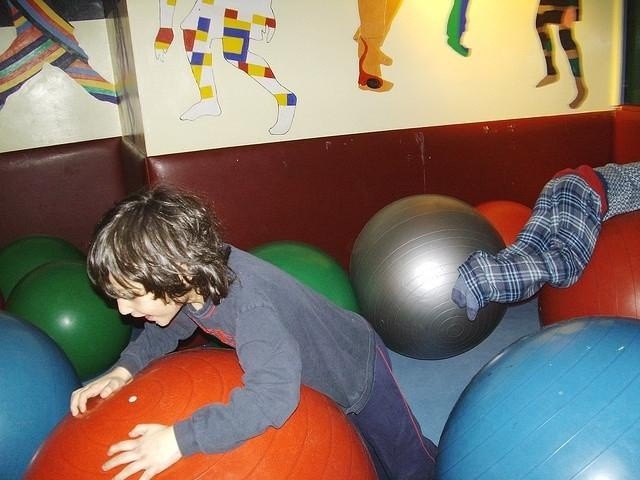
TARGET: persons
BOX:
[451,159,639,327]
[66,184,440,478]
